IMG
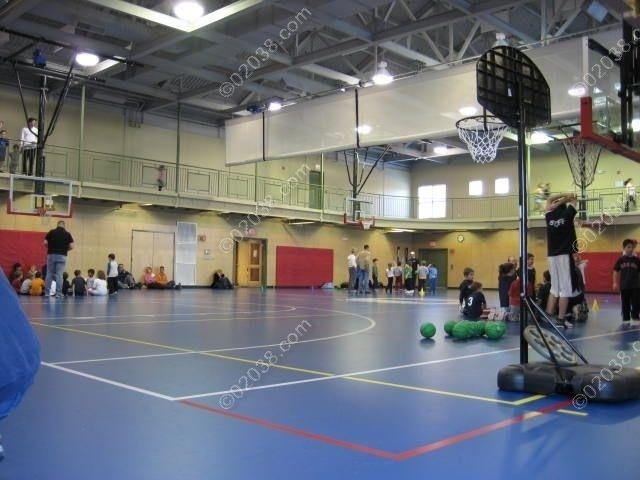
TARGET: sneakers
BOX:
[488,307,497,321]
[580,303,588,321]
[540,312,553,327]
[498,307,508,321]
[554,319,573,329]
[572,304,582,322]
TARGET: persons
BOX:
[356,244,372,294]
[627,177,638,211]
[620,240,639,316]
[212,269,235,289]
[7,143,20,174]
[117,264,142,288]
[529,182,545,215]
[8,252,118,296]
[371,258,381,291]
[457,266,474,307]
[0,264,42,459]
[461,281,487,321]
[625,179,630,213]
[157,164,166,190]
[497,252,589,320]
[155,265,182,289]
[143,266,161,290]
[385,251,439,296]
[19,116,38,176]
[0,129,9,173]
[612,239,640,322]
[0,120,4,130]
[543,192,579,328]
[545,182,552,200]
[42,220,75,299]
[346,247,356,294]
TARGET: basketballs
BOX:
[420,322,436,338]
[443,321,507,340]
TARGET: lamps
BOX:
[369,57,395,88]
